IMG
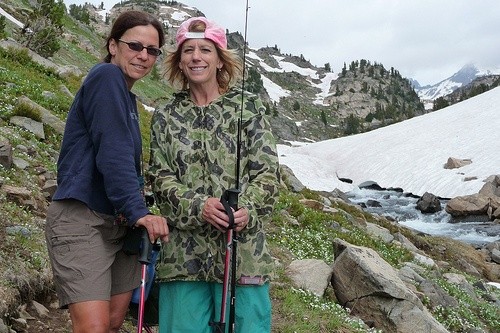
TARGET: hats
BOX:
[175,17,227,53]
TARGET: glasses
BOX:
[118,39,163,57]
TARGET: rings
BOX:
[242,222,244,227]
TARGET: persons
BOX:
[147,16,281,333]
[45,11,169,333]
